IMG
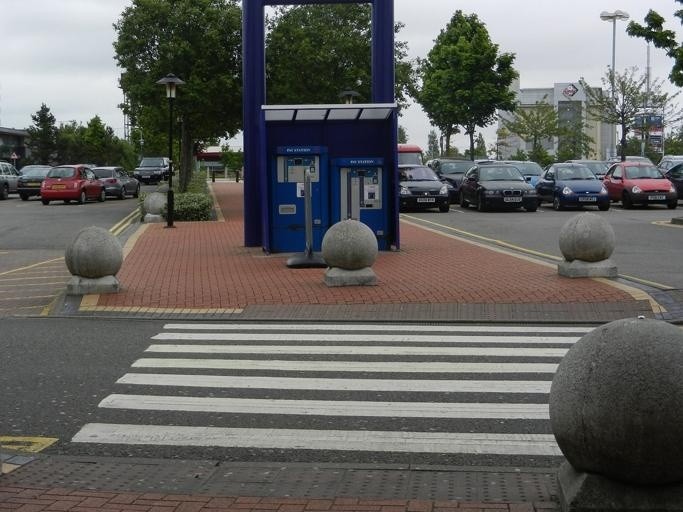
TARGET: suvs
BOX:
[0,160,25,200]
[133,156,174,185]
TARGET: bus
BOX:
[397,143,425,166]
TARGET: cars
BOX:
[397,152,682,214]
[20,163,143,206]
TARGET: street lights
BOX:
[598,9,630,160]
[176,112,183,176]
[336,87,360,104]
[138,138,146,156]
[154,72,188,229]
